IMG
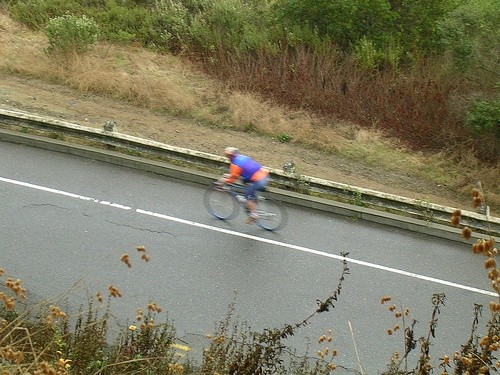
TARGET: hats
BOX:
[223,147,239,155]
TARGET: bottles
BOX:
[236,195,246,203]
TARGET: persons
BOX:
[218,147,271,226]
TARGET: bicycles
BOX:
[205,175,288,232]
